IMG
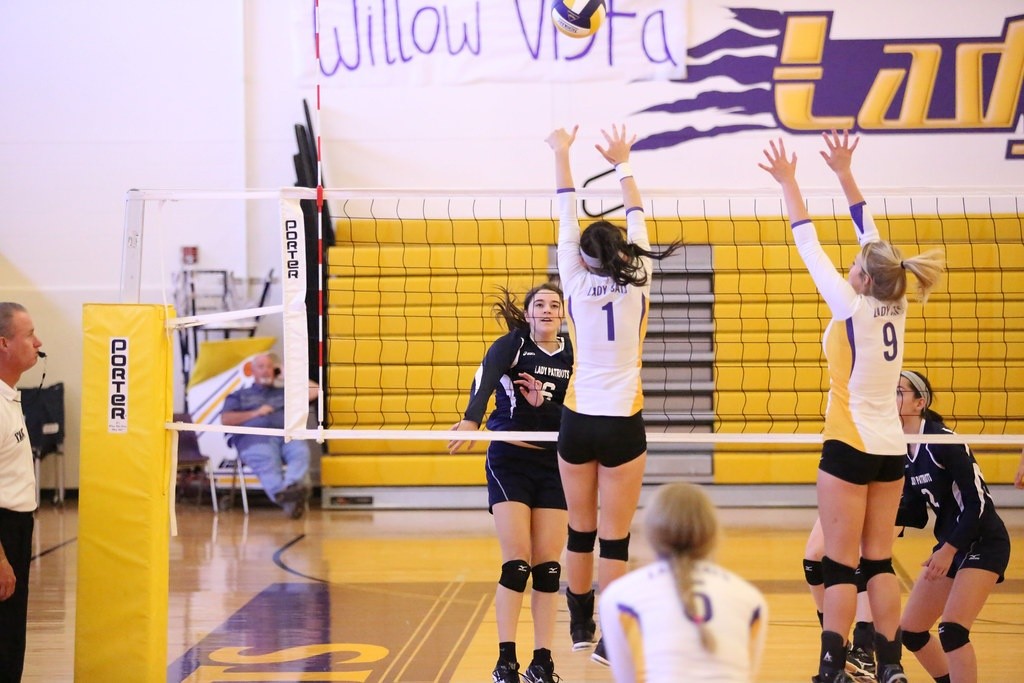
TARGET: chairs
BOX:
[172,414,253,515]
[18,385,67,506]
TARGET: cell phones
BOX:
[273,368,282,376]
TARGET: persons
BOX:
[757,126,943,683]
[0,303,44,683]
[803,370,1011,683]
[448,283,575,683]
[220,353,312,518]
[599,482,770,683]
[545,123,653,667]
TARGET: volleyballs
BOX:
[551,0,607,38]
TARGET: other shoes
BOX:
[590,638,612,670]
[572,630,596,651]
[874,663,908,683]
[491,659,520,683]
[274,485,308,500]
[283,497,304,519]
[812,665,856,683]
[525,666,563,683]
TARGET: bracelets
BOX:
[615,162,633,182]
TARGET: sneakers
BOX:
[844,648,877,679]
